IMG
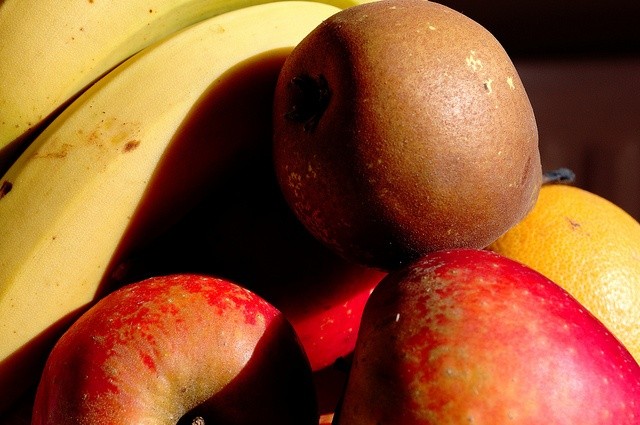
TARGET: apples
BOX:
[331,247,640,425]
[30,274,321,425]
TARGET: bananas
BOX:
[0,1,344,424]
[0,1,375,174]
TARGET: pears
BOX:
[273,0,576,272]
